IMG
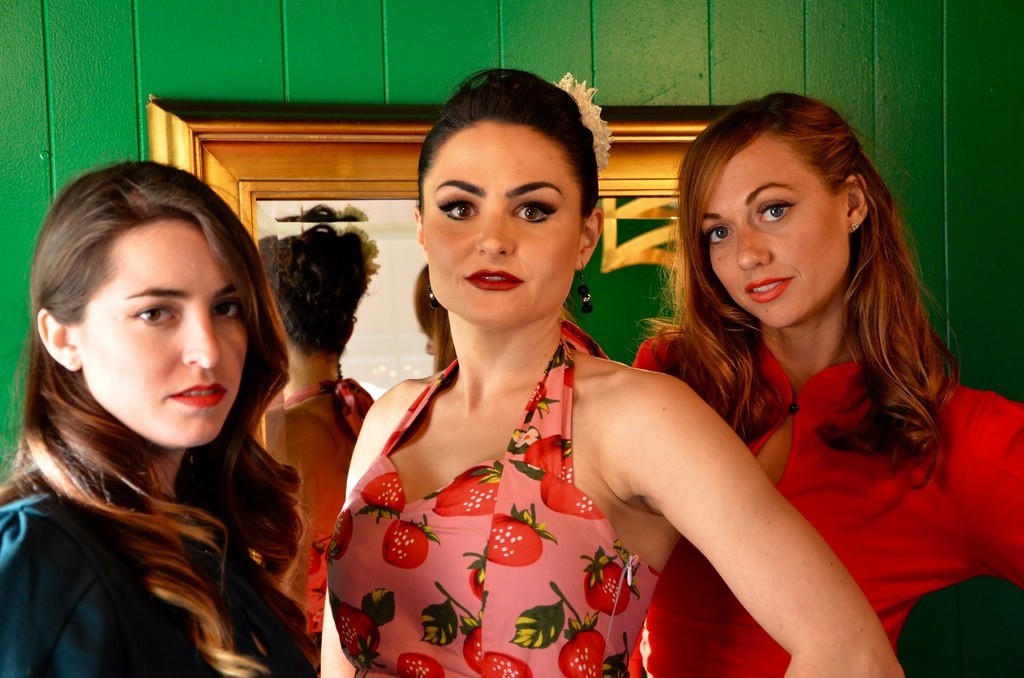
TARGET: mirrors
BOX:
[145,96,740,678]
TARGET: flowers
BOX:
[555,71,612,170]
[337,224,383,285]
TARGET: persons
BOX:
[325,67,902,678]
[630,92,1024,678]
[1,162,318,678]
[258,223,375,676]
[413,261,457,373]
